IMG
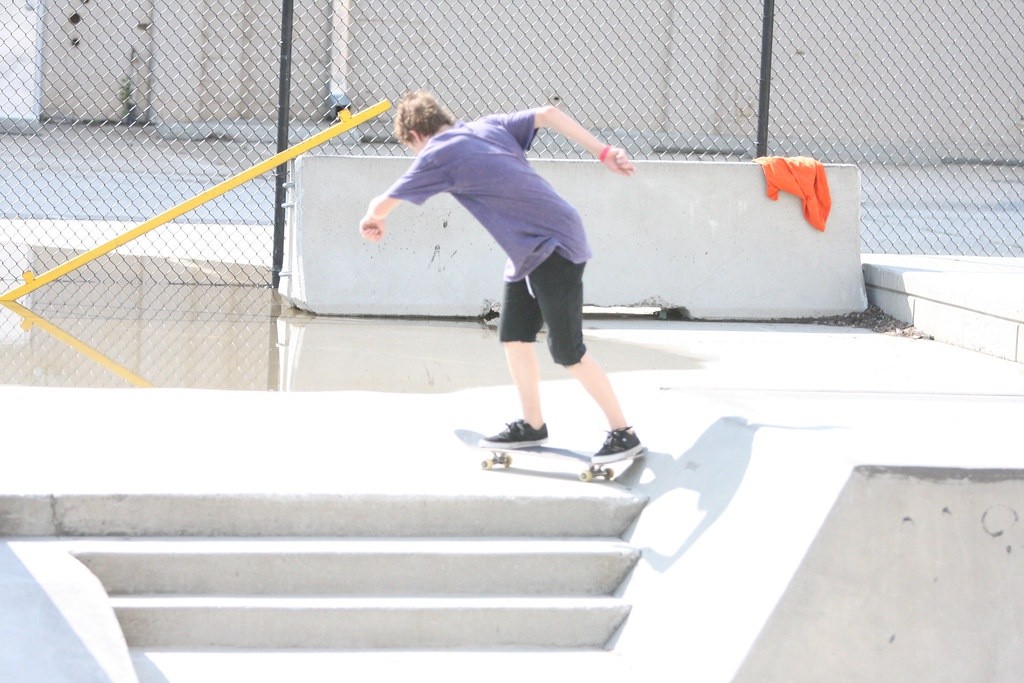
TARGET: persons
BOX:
[358,92,644,464]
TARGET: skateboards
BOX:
[453,427,648,483]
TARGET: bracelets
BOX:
[598,146,611,163]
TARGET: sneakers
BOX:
[478,418,550,449]
[591,425,644,464]
